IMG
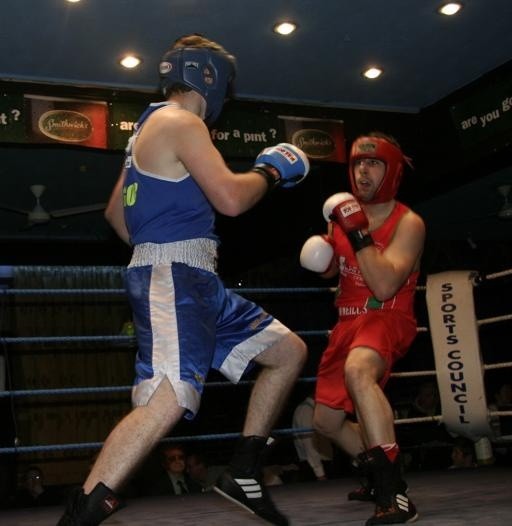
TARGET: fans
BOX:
[0,182,112,236]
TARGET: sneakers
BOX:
[348,488,419,526]
[56,482,127,525]
[212,469,291,526]
[355,478,412,505]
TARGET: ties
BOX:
[175,479,188,495]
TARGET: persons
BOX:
[164,378,512,502]
[54,34,312,524]
[3,467,58,508]
[299,132,427,524]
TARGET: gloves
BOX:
[322,192,374,254]
[299,234,335,275]
[251,141,311,189]
